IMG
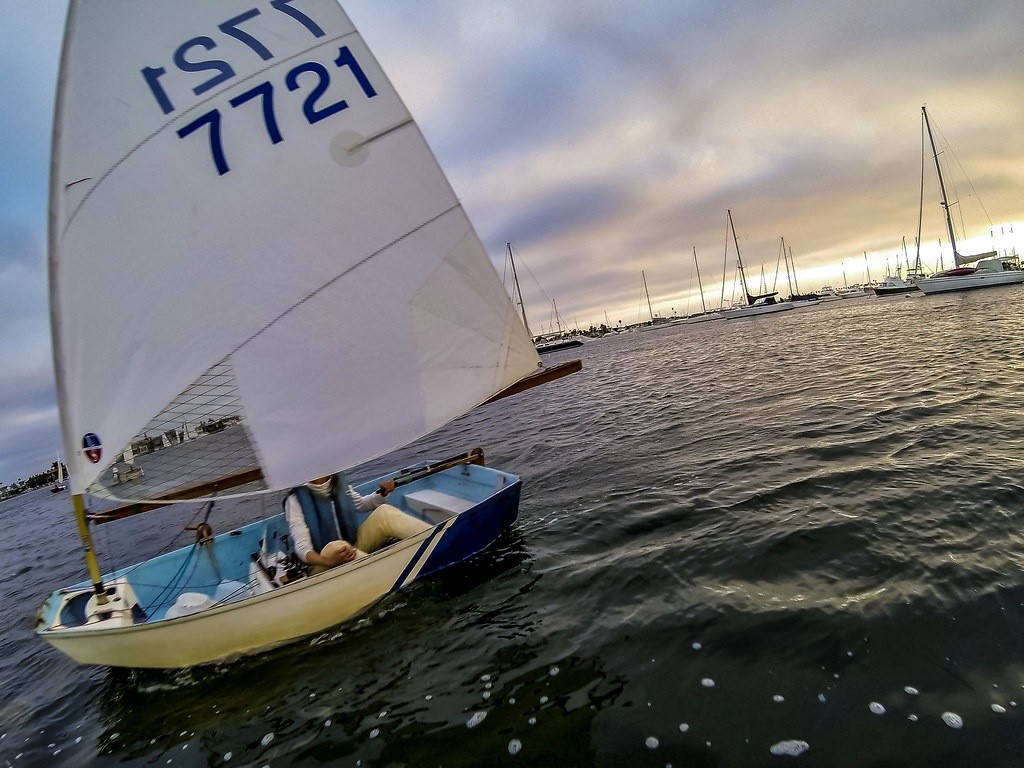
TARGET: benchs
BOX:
[402,488,480,525]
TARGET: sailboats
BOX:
[912,105,1024,294]
[36,2,543,666]
[638,270,690,332]
[771,237,822,308]
[161,421,207,450]
[501,240,583,355]
[873,236,935,297]
[840,252,876,298]
[720,210,795,318]
[671,247,725,324]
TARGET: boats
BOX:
[811,284,842,302]
[50,459,67,493]
[113,465,143,485]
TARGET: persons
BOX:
[282,470,434,576]
